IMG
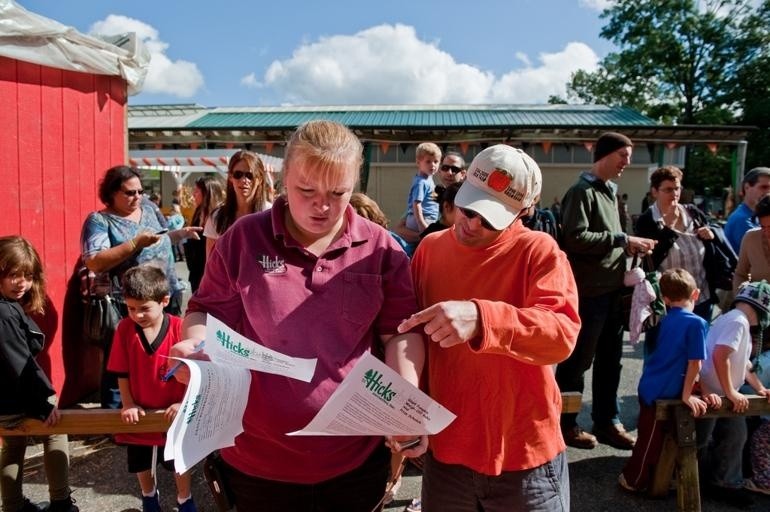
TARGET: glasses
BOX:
[125,190,143,195]
[441,165,462,173]
[233,171,253,179]
[459,208,493,229]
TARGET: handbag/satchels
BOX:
[621,270,667,332]
[81,295,122,344]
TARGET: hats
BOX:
[593,132,633,162]
[454,144,542,230]
[731,279,770,330]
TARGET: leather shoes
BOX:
[562,425,597,448]
[592,423,636,449]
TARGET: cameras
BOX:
[637,244,650,254]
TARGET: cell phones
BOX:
[152,228,168,235]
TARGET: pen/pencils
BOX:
[163,341,205,381]
